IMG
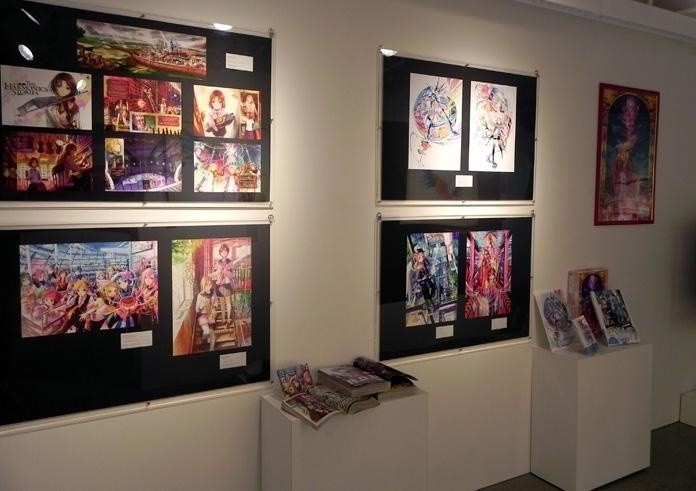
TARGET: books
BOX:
[571,314,600,356]
[589,289,641,348]
[274,357,418,432]
[533,288,579,354]
[568,267,608,340]
